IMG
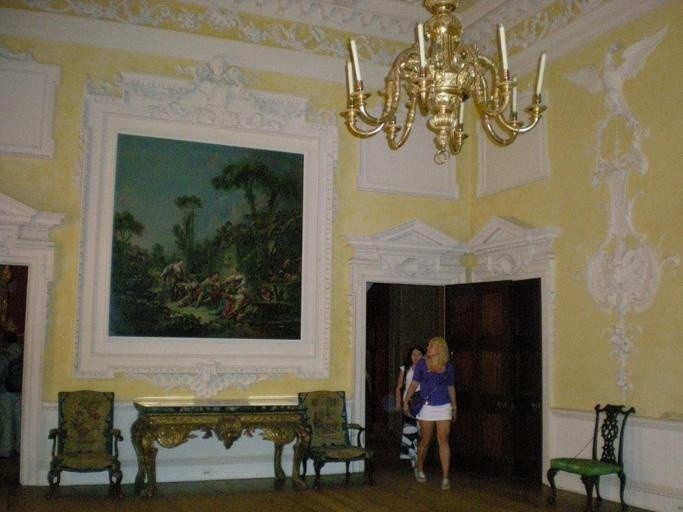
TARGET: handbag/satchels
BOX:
[400,364,408,403]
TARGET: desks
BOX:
[130,398,312,497]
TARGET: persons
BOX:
[159,257,301,318]
[404,337,457,488]
[395,346,424,479]
[1,331,23,459]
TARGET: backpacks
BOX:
[2,350,23,393]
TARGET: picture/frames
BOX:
[75,70,335,379]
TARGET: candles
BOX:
[350,38,362,80]
[536,50,546,96]
[417,20,426,74]
[499,23,508,79]
[512,76,517,113]
[347,56,354,100]
[459,101,465,124]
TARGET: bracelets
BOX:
[451,405,456,409]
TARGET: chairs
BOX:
[296,390,373,484]
[47,390,123,498]
[547,403,636,512]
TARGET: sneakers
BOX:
[414,469,427,482]
[441,479,451,489]
